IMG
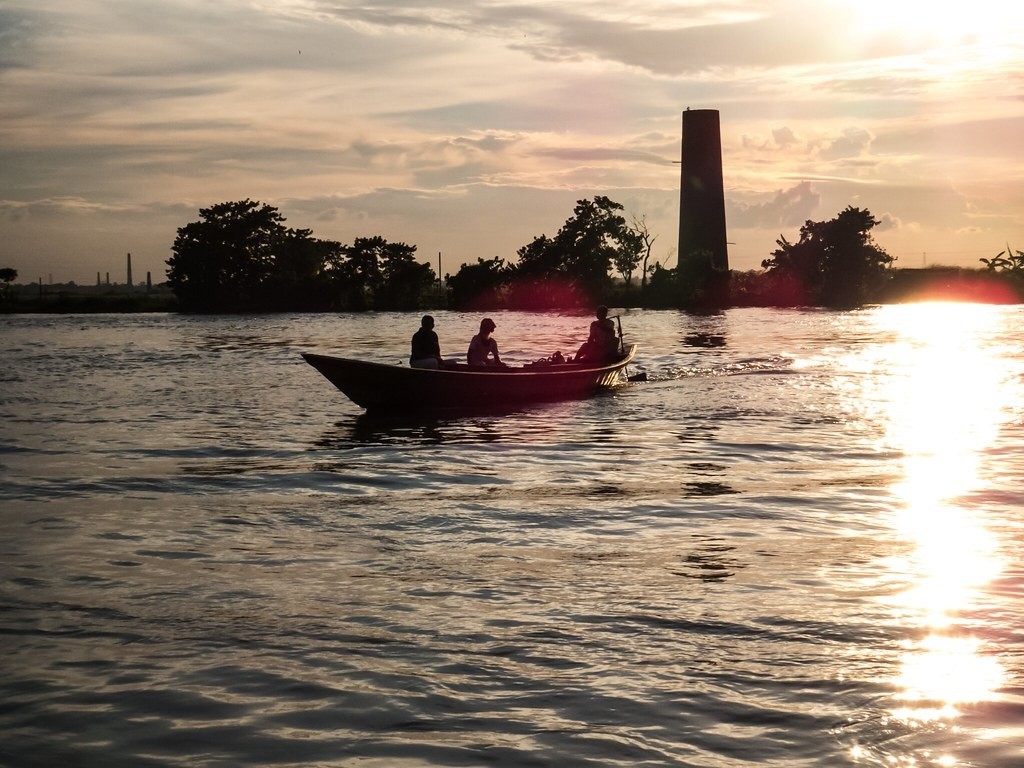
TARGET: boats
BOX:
[299,342,638,410]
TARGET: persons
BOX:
[467,318,504,367]
[409,315,442,370]
[568,305,619,364]
